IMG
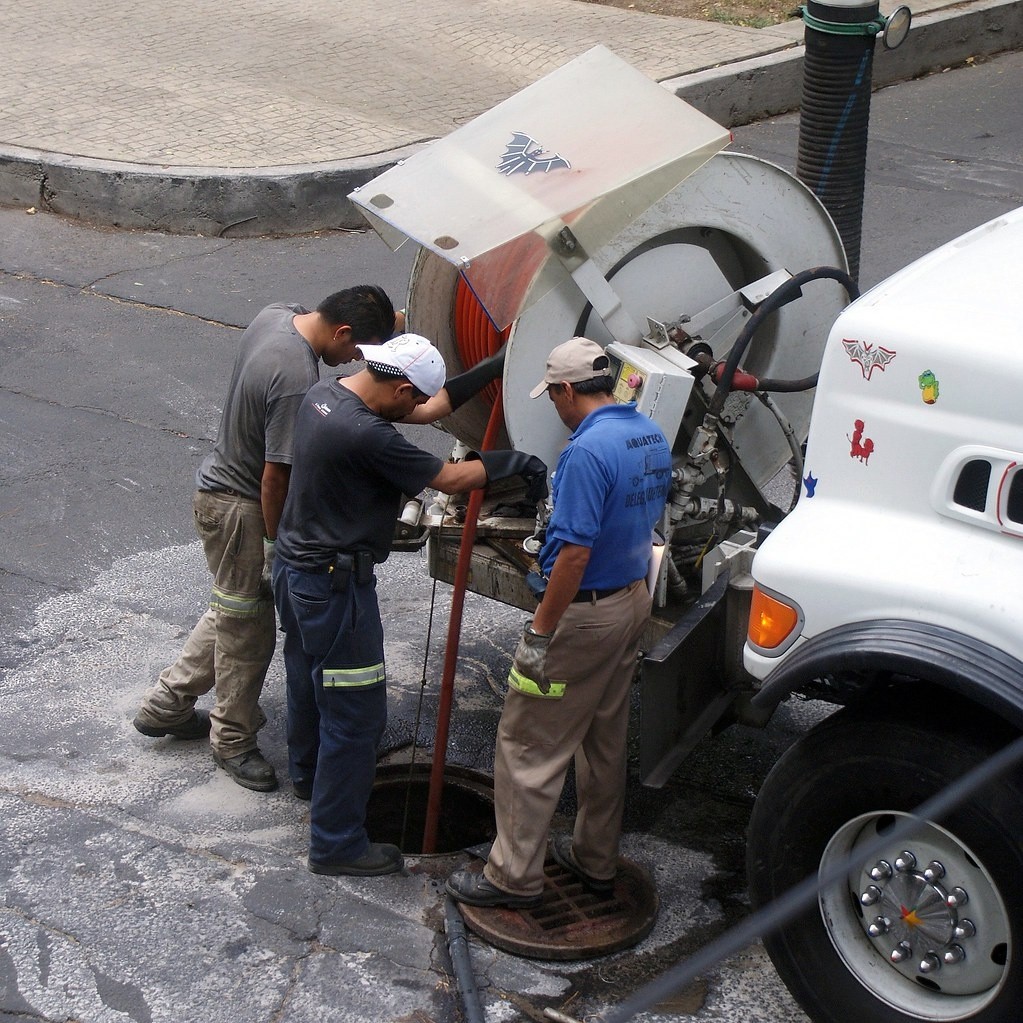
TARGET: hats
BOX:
[529,336,612,399]
[354,333,446,397]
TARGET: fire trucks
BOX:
[342,32,1022,1020]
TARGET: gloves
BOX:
[464,448,549,503]
[516,619,553,696]
[443,342,509,411]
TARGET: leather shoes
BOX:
[308,842,405,876]
[294,780,313,799]
[552,835,617,893]
[446,870,542,910]
[214,747,278,790]
[133,708,212,738]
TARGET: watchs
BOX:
[523,627,552,647]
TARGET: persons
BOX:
[131,283,411,789]
[267,332,552,876]
[444,336,675,907]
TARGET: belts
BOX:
[222,488,250,498]
[570,586,629,602]
